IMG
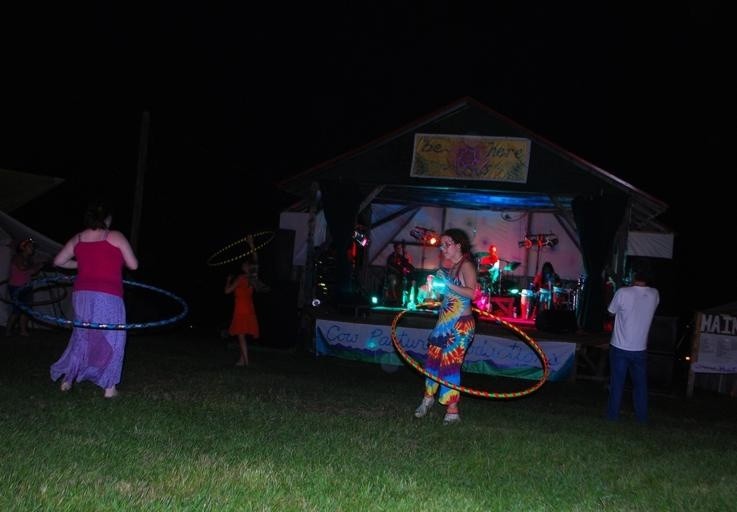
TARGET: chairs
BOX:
[415,397,435,418]
[443,413,459,425]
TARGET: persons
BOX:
[50,198,139,398]
[413,228,477,427]
[224,232,260,367]
[386,242,406,306]
[489,242,500,283]
[532,262,562,289]
[5,239,46,336]
[604,262,660,423]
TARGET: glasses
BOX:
[518,233,559,284]
[410,226,437,285]
[351,231,368,319]
